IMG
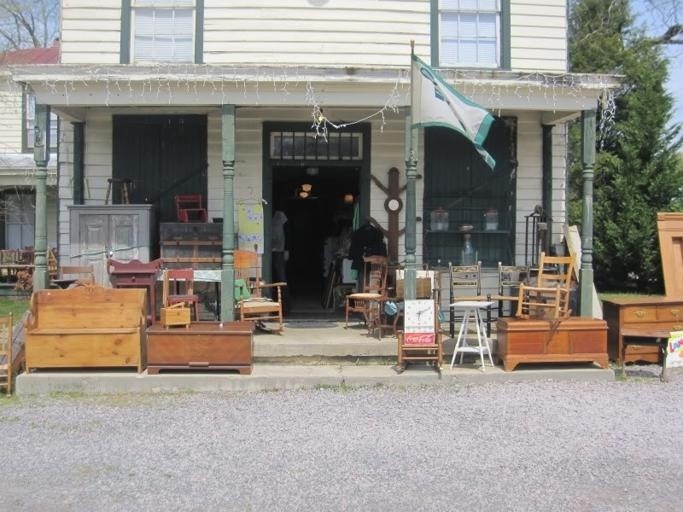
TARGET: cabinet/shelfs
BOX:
[107,259,160,324]
[602,301,683,367]
[67,205,150,288]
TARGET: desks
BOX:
[447,301,497,372]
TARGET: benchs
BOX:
[22,288,148,374]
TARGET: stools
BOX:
[105,177,132,203]
[160,302,191,330]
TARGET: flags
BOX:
[411,52,497,172]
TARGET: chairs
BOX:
[0,247,56,283]
[235,266,287,332]
[62,264,95,284]
[394,299,445,374]
[0,312,25,398]
[345,255,388,340]
[447,205,577,339]
[162,268,200,319]
[175,195,208,222]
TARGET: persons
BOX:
[272,210,291,311]
